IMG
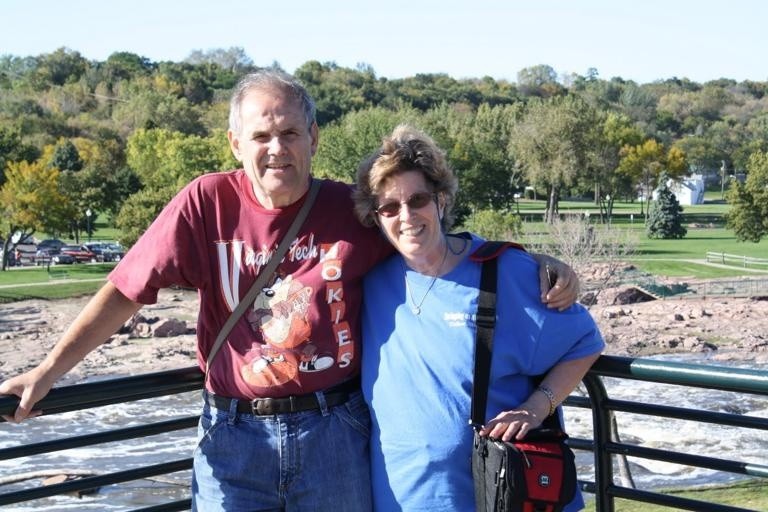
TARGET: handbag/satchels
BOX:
[468,422,580,512]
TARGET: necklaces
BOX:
[398,243,447,316]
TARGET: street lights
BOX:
[720,165,725,200]
[514,193,521,214]
[85,208,91,243]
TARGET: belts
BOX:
[200,372,364,417]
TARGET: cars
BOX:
[0,232,126,266]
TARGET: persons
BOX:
[0,70,580,512]
[352,125,606,512]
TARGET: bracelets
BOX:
[536,387,558,419]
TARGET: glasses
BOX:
[373,187,440,218]
